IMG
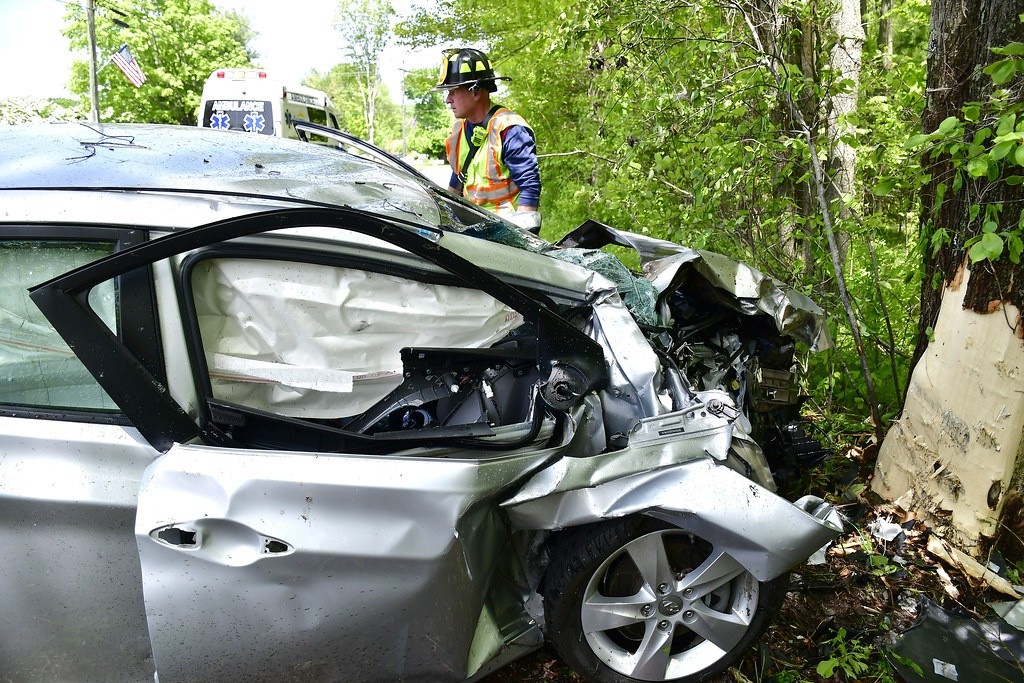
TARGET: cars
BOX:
[0,115,846,683]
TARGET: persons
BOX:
[437,48,542,239]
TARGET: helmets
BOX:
[436,48,512,92]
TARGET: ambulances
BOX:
[196,68,347,152]
